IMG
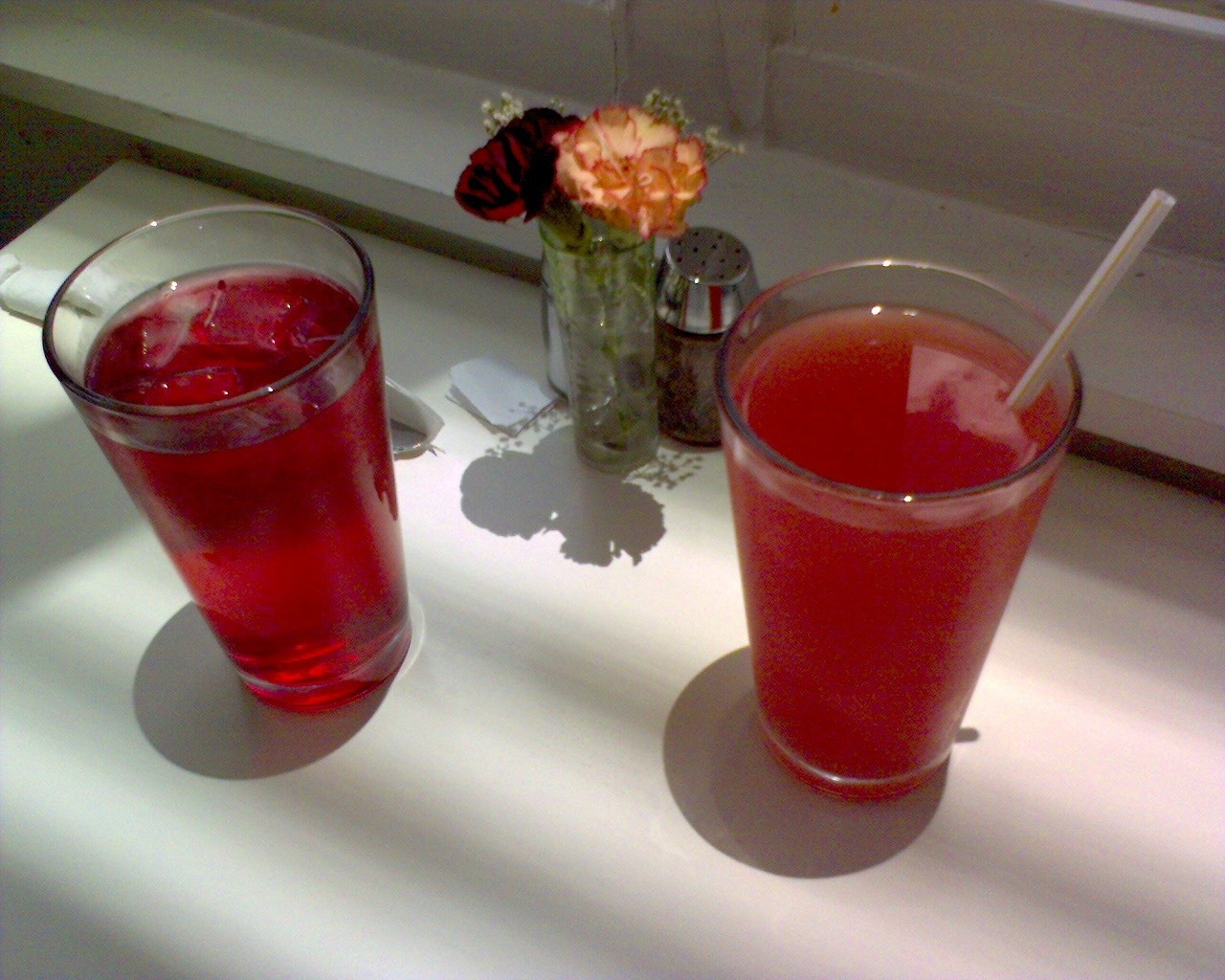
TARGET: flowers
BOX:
[453,85,750,245]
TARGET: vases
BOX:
[538,241,661,470]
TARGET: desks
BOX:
[0,159,1225,980]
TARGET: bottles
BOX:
[640,221,772,448]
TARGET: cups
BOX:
[41,199,417,708]
[712,255,1086,802]
